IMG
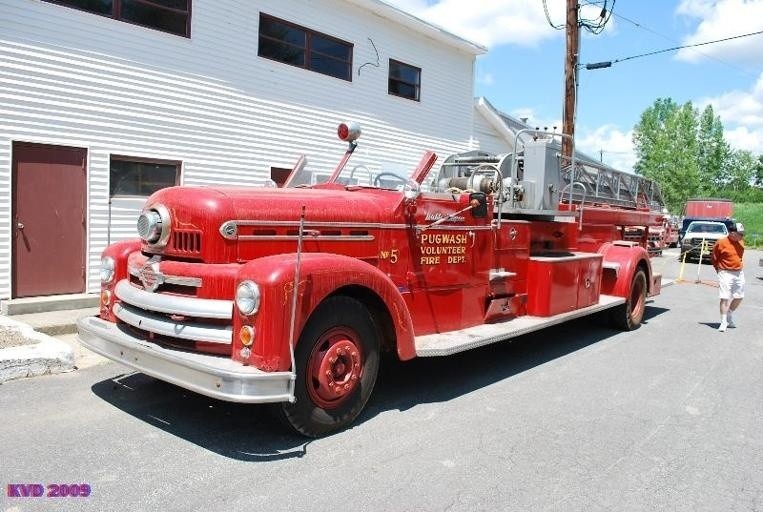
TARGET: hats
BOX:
[728,223,744,232]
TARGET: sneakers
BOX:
[718,319,737,332]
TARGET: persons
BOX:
[710,222,746,332]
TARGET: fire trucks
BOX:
[73,125,681,438]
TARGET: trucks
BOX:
[680,197,736,265]
